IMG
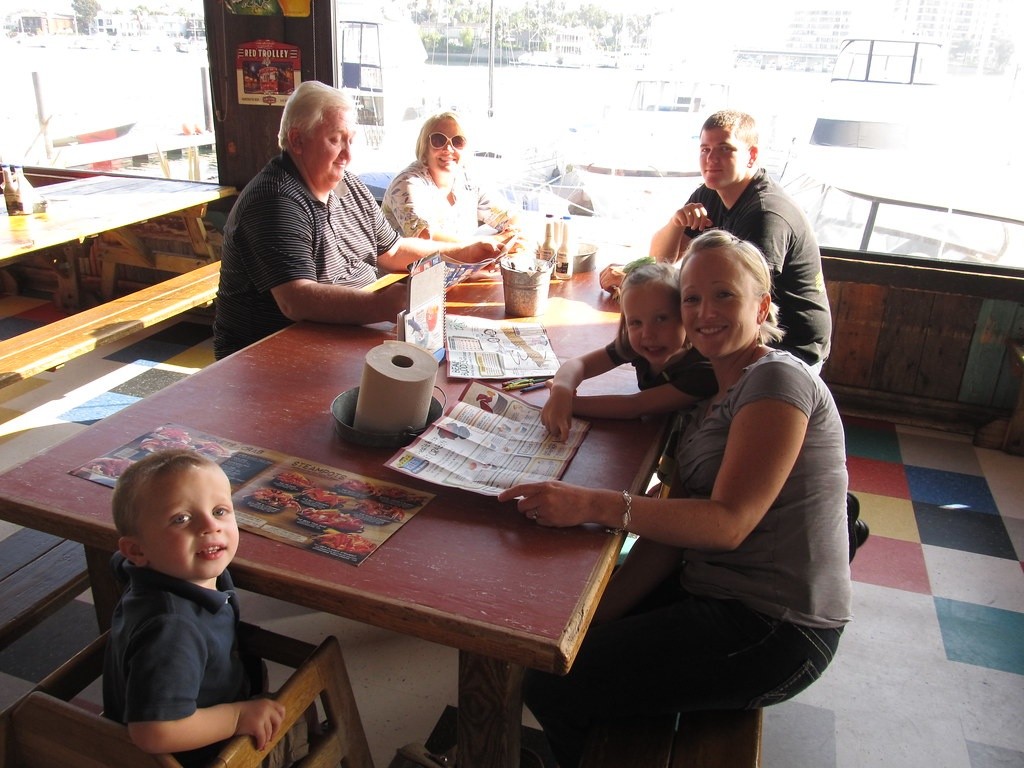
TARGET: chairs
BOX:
[0,623,377,768]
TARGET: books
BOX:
[406,261,446,354]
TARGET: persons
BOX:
[380,112,528,254]
[542,264,869,562]
[600,111,832,373]
[498,231,853,768]
[101,450,329,768]
[213,81,506,360]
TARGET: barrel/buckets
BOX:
[500,259,555,318]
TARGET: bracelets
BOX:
[605,490,632,535]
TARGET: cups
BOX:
[33,202,46,214]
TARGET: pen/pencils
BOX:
[501,377,545,391]
[520,383,545,393]
[510,262,515,270]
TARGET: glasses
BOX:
[428,132,468,150]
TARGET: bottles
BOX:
[0,164,23,216]
[540,215,572,279]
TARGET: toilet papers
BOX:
[353,340,439,433]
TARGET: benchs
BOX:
[0,527,88,635]
[0,261,221,390]
[657,482,762,768]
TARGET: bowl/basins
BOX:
[330,386,447,449]
[573,242,598,273]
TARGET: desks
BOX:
[0,252,672,768]
[0,176,236,304]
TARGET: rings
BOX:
[533,508,538,519]
[686,211,692,217]
